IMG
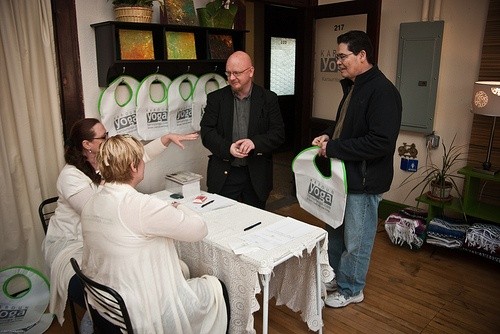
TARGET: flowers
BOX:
[206,0,238,17]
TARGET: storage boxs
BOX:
[167,180,200,198]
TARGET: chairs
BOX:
[69,257,135,334]
[39,196,88,334]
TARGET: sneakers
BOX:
[324,278,338,290]
[325,289,364,307]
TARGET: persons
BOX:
[200,51,286,210]
[311,30,403,308]
[41,118,199,334]
[80,133,231,334]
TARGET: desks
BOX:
[148,189,335,334]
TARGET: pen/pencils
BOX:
[202,200,214,207]
[244,222,261,231]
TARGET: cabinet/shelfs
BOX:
[90,20,251,88]
[384,163,500,263]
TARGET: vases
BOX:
[197,8,238,29]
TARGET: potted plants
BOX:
[394,131,468,224]
[111,0,166,23]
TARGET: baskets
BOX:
[114,6,153,23]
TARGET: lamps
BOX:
[471,80,500,171]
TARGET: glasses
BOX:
[225,66,252,77]
[336,48,365,61]
[86,132,108,141]
